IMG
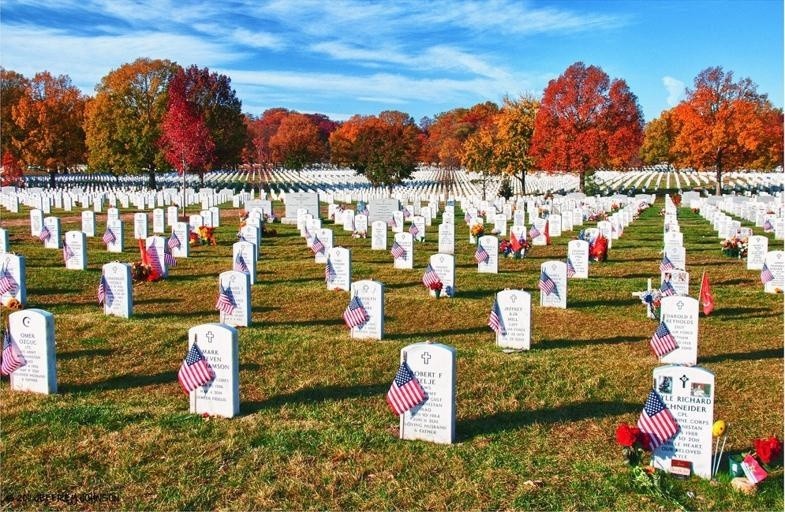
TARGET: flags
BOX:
[215,285,234,314]
[177,342,212,397]
[487,299,499,333]
[0,269,11,295]
[637,389,680,451]
[701,273,715,316]
[650,322,679,358]
[386,362,426,416]
[38,208,775,303]
[0,328,21,376]
[343,296,366,329]
